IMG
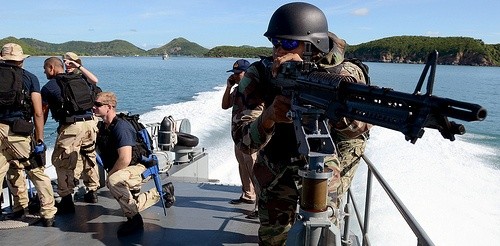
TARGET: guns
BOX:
[34,144,45,167]
[137,128,169,216]
[275,60,489,144]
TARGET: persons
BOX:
[232,2,372,246]
[39,56,100,214]
[62,51,105,191]
[93,91,176,238]
[0,42,58,227]
[222,59,265,219]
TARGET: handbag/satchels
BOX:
[11,119,34,136]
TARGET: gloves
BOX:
[34,139,47,166]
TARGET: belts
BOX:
[76,117,94,122]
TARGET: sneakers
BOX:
[14,205,39,219]
[162,182,177,208]
[42,218,56,227]
[84,190,98,202]
[116,212,143,236]
[56,195,75,213]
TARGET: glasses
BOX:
[93,101,115,108]
[270,38,299,51]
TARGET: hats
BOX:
[227,59,251,74]
[61,51,82,66]
[0,43,30,61]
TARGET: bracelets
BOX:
[77,66,81,70]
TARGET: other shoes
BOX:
[247,211,259,219]
[231,197,255,205]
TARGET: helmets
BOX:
[263,2,330,54]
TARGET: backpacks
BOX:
[51,73,93,115]
[0,62,25,110]
[99,112,153,159]
